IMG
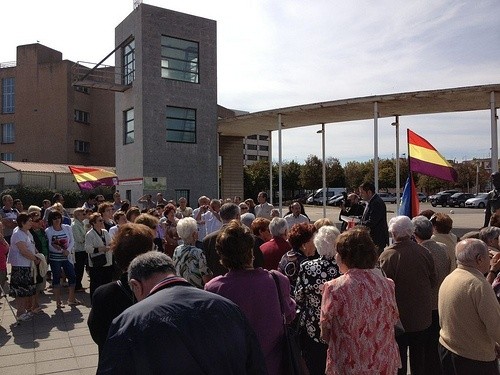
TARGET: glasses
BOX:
[96,219,105,223]
[53,217,61,220]
[98,200,105,202]
[33,218,42,223]
[242,207,248,210]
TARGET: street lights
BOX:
[316,123,327,218]
[390,116,401,216]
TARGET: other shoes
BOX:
[16,313,33,321]
[56,301,65,308]
[67,298,83,305]
[30,306,44,314]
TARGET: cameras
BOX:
[334,192,352,207]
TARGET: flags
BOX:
[69,166,119,191]
[407,129,459,182]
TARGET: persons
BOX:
[0,181,500,322]
[95,250,268,375]
[204,218,297,375]
[320,225,402,375]
[438,238,500,375]
[378,215,439,375]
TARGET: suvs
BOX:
[428,190,458,208]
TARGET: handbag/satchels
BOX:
[281,331,311,375]
[91,254,107,267]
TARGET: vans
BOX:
[306,188,346,204]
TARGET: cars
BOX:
[327,194,365,207]
[377,192,427,204]
[464,193,488,209]
[446,193,476,208]
[474,193,487,196]
